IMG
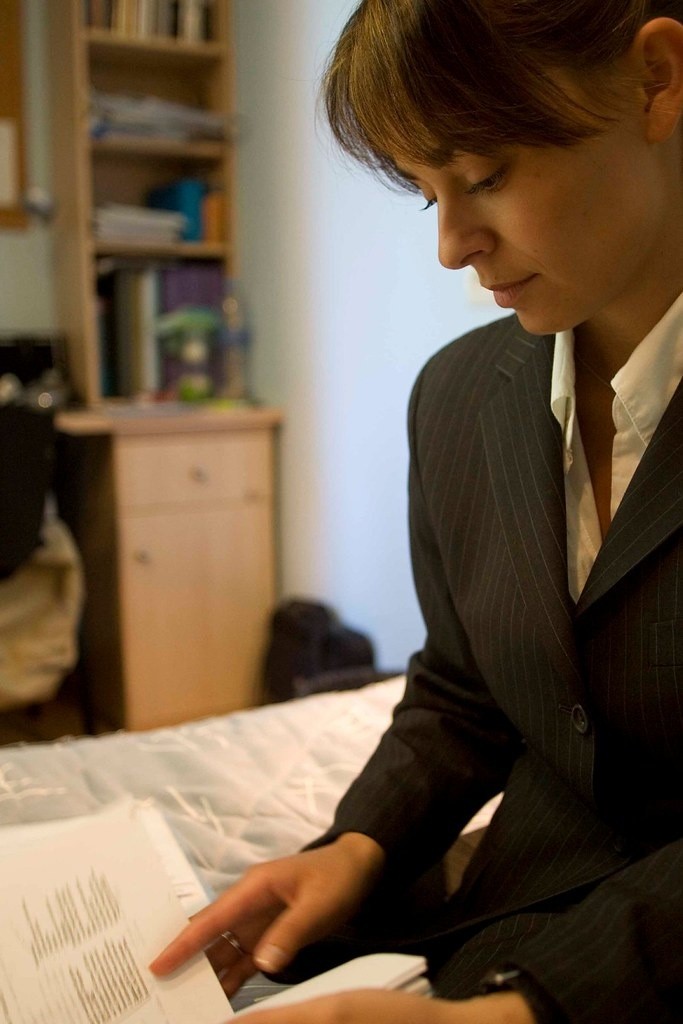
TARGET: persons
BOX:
[149,0,683,1024]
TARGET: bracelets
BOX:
[481,967,555,1024]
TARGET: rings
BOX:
[221,931,244,955]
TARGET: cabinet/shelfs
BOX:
[39,0,282,731]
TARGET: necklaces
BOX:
[575,348,615,394]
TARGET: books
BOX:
[91,203,184,240]
[84,0,208,41]
[0,810,433,1024]
[95,264,225,398]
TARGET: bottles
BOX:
[213,284,247,397]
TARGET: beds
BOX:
[0,668,526,1024]
